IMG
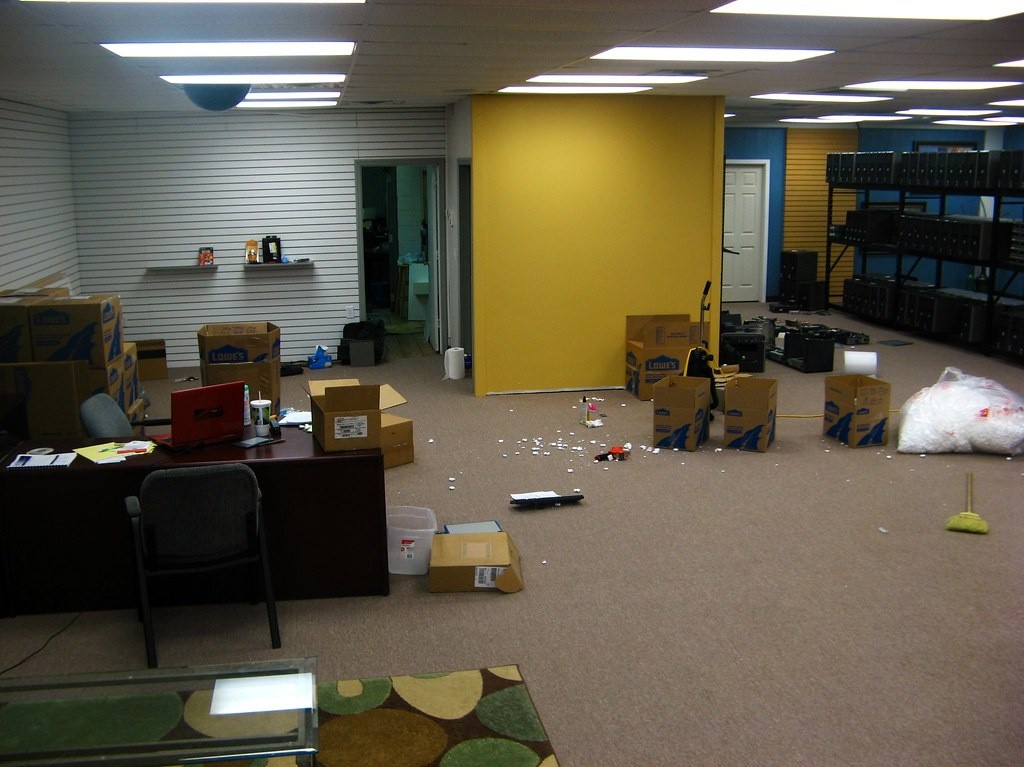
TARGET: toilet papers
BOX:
[444,347,465,380]
[842,350,877,376]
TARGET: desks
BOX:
[0,656,319,767]
[0,425,391,621]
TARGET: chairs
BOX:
[80,393,172,439]
[126,463,281,668]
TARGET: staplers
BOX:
[270,418,282,436]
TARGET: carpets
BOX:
[0,664,564,767]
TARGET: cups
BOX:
[250,400,271,436]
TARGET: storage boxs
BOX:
[197,322,282,417]
[385,505,438,576]
[823,374,890,447]
[626,314,777,453]
[428,534,524,593]
[301,378,407,453]
[0,273,168,443]
[381,412,414,470]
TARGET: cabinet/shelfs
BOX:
[825,184,1024,369]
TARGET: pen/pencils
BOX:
[100,446,121,452]
[117,449,147,454]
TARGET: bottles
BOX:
[248,250,256,262]
[581,396,588,421]
[244,384,251,425]
[587,402,597,421]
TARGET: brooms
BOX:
[947,470,987,535]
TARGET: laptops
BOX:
[150,381,245,451]
